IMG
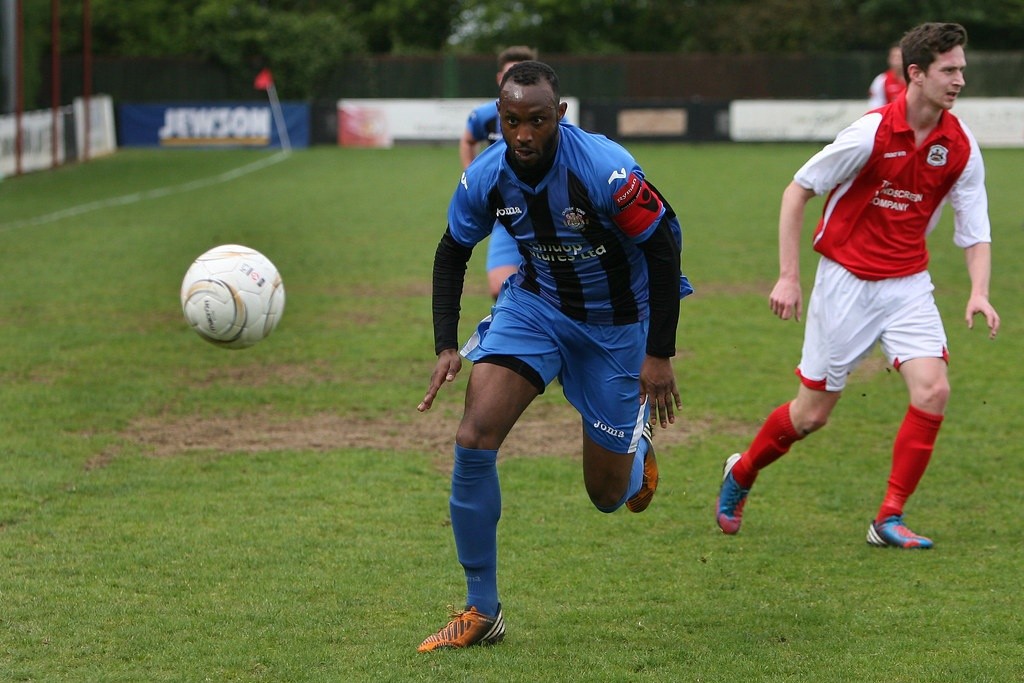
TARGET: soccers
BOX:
[178,244,286,352]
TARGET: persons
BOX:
[868,44,906,108]
[459,46,539,300]
[715,22,1003,547]
[415,60,691,653]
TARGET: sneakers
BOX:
[625,421,658,513]
[716,452,751,535]
[866,514,934,550]
[418,602,505,653]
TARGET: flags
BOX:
[256,71,271,89]
